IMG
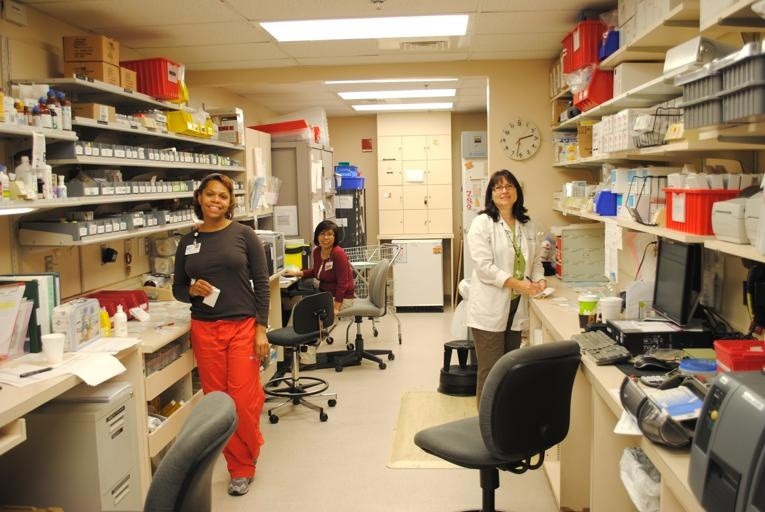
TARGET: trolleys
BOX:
[328,241,403,351]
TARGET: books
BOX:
[534,286,555,298]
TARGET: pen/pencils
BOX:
[526,276,533,283]
[20,367,52,377]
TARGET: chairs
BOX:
[324,255,396,372]
[142,389,239,511]
[264,289,338,425]
[412,336,584,512]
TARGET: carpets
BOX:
[386,388,545,473]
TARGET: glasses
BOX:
[492,183,514,193]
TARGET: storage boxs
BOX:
[547,1,765,239]
[57,33,215,140]
[331,160,365,194]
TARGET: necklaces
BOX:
[497,220,523,279]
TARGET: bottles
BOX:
[0,155,70,199]
[1,88,72,131]
[114,303,129,335]
[134,108,165,115]
[100,305,113,336]
[578,294,599,328]
[602,270,622,296]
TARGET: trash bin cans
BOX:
[618,447,661,512]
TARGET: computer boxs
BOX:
[605,318,712,356]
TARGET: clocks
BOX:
[497,115,544,162]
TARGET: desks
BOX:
[0,334,155,512]
[376,234,456,314]
[520,269,729,512]
[108,267,284,475]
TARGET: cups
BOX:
[40,333,66,361]
[600,296,624,324]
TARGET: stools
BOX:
[433,338,479,397]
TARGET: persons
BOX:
[466,170,548,416]
[280,221,354,326]
[171,174,269,497]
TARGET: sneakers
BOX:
[226,475,253,495]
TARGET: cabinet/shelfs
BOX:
[0,76,246,250]
[550,3,765,267]
[251,139,339,272]
[374,110,453,240]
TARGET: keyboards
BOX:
[571,329,631,365]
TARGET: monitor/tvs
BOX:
[653,235,705,327]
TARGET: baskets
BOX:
[660,183,745,238]
[118,56,182,101]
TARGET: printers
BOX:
[688,366,765,512]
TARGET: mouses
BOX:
[633,356,668,371]
